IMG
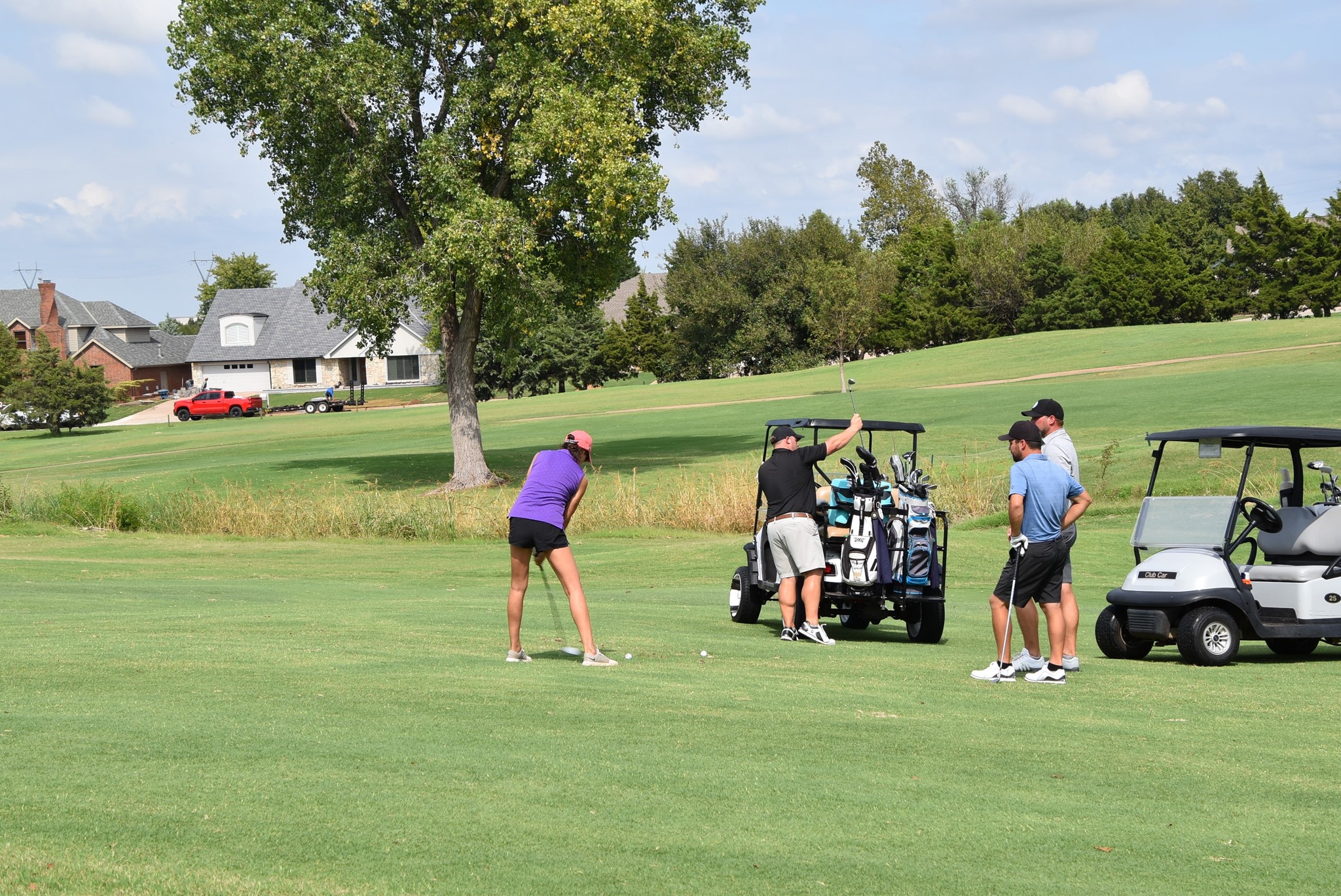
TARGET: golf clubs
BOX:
[989,540,1024,686]
[847,378,866,451]
[839,445,891,491]
[897,449,938,498]
[1306,460,1341,504]
[532,551,584,657]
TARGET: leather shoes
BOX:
[767,513,814,525]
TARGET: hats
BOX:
[1021,399,1064,419]
[998,420,1042,441]
[204,376,209,380]
[564,430,593,465]
[771,426,805,445]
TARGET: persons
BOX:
[201,377,208,389]
[325,388,334,402]
[758,412,862,646]
[506,430,619,667]
[970,421,1091,684]
[1009,398,1079,672]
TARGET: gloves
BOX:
[1010,532,1028,556]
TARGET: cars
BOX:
[0,402,84,430]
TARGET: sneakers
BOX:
[505,649,533,662]
[798,621,836,645]
[582,649,618,667]
[970,661,1015,681]
[1025,665,1066,684]
[781,627,799,641]
[1045,654,1080,670]
[1013,648,1045,671]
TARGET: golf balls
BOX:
[700,651,707,656]
[625,653,632,659]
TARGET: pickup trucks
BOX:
[173,390,263,422]
